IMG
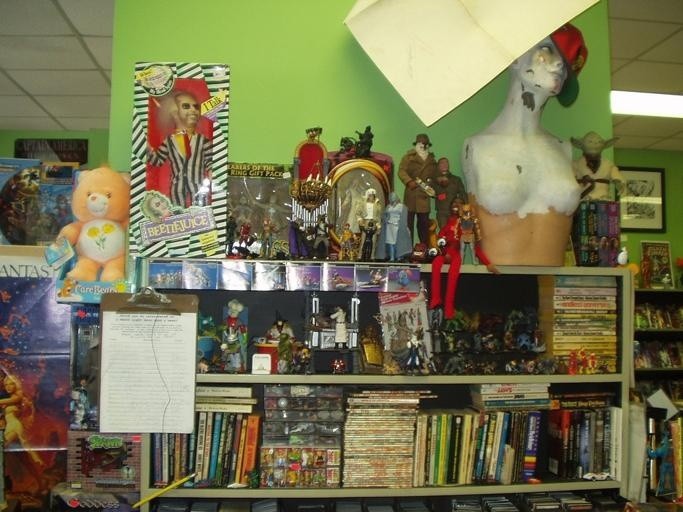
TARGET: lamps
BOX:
[610,88,683,119]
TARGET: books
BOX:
[414,383,622,488]
[342,389,437,487]
[153,387,260,487]
[538,275,618,374]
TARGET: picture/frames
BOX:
[617,167,667,233]
[642,240,675,290]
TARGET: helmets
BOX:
[464,203,472,211]
[224,327,238,343]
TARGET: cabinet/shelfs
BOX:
[635,289,683,512]
[138,259,633,512]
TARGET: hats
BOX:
[553,24,588,109]
[413,133,431,145]
[277,333,289,338]
[272,310,288,326]
[367,188,376,195]
[229,299,243,312]
[398,310,407,317]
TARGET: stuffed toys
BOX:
[571,132,620,205]
[56,166,131,282]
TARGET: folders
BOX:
[96,286,198,433]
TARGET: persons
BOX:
[145,90,212,207]
[266,308,312,374]
[227,209,354,261]
[1,374,43,466]
[436,157,468,229]
[330,304,349,349]
[376,193,413,261]
[463,21,589,269]
[398,133,435,247]
[430,197,500,319]
[209,300,246,373]
[356,189,381,260]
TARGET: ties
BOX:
[183,131,192,160]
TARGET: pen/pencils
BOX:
[132,471,198,508]
